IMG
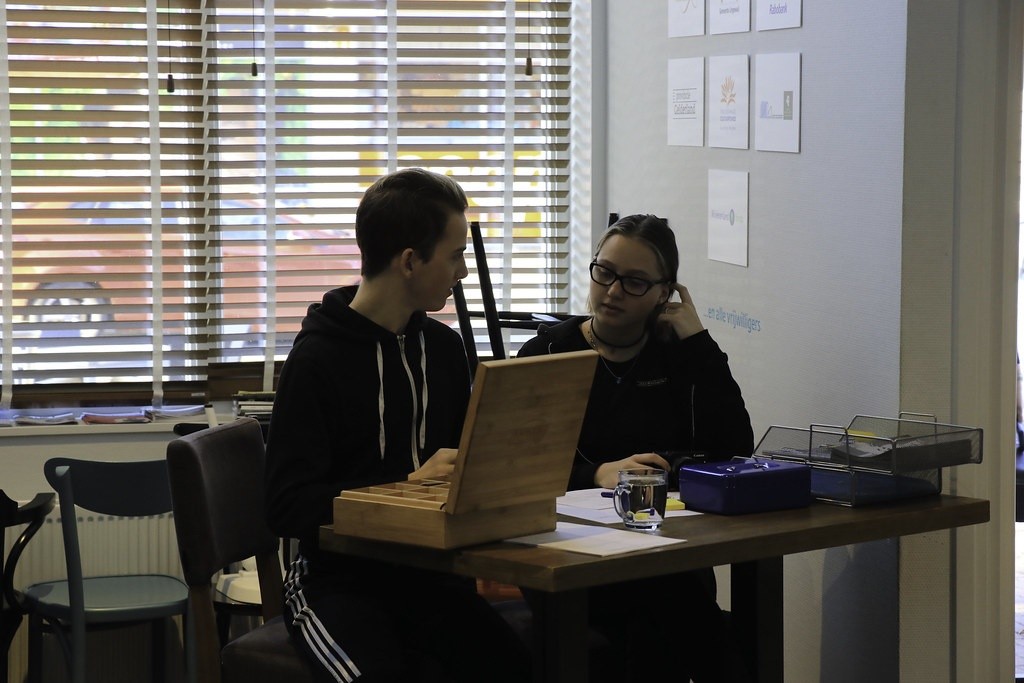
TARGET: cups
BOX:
[614,468,668,532]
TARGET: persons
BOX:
[263,171,534,683]
[518,214,755,683]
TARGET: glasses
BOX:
[589,260,674,296]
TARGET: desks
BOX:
[319,495,992,683]
[0,401,271,683]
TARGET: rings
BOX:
[665,308,669,314]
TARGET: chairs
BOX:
[173,422,279,647]
[166,418,332,683]
[0,488,56,683]
[22,457,190,683]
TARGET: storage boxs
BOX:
[332,349,599,549]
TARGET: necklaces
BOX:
[589,326,638,385]
[591,318,646,353]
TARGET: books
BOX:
[232,391,277,421]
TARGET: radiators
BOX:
[5,500,223,683]
